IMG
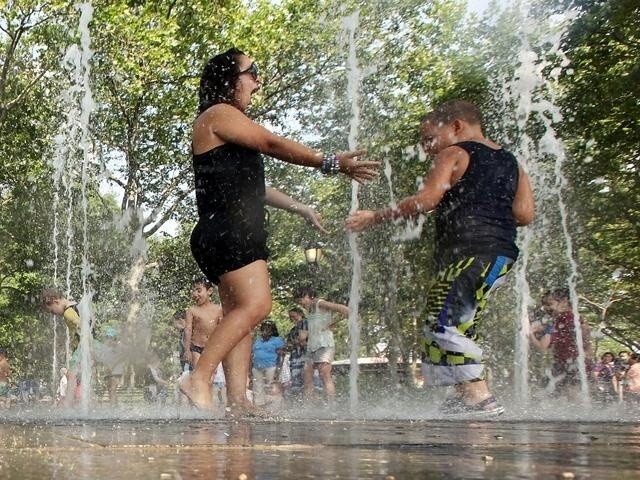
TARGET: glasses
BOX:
[240,61,257,80]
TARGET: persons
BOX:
[42,285,170,412]
[180,278,351,411]
[346,99,535,422]
[0,348,81,411]
[529,286,640,408]
[176,48,383,423]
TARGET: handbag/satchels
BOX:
[279,352,292,389]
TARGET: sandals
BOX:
[440,395,505,417]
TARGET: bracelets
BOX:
[322,151,340,175]
